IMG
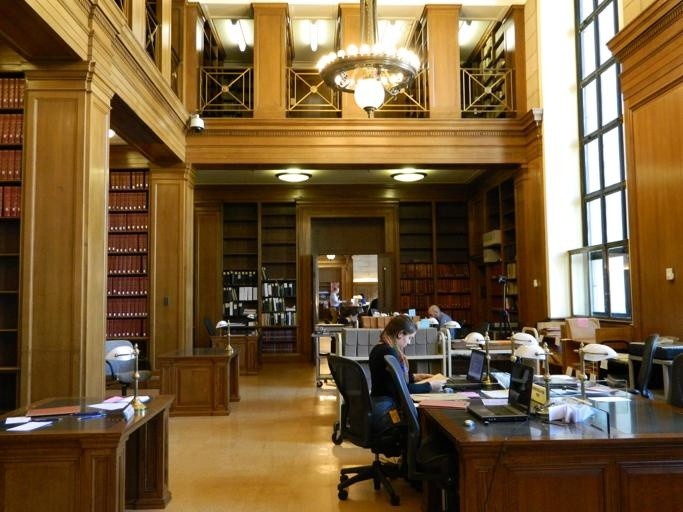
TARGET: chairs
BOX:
[327,354,401,506]
[200,317,215,346]
[637,334,658,394]
[384,355,451,511]
[560,318,628,375]
[668,353,682,407]
[103,339,152,413]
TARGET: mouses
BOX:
[443,387,453,393]
[464,420,474,426]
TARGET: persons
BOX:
[368,314,446,490]
[360,293,366,304]
[329,287,342,324]
[428,304,455,340]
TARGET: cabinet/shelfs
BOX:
[107,167,147,362]
[396,198,473,327]
[338,328,446,376]
[0,71,24,408]
[476,176,524,332]
[310,331,338,387]
[221,198,298,357]
[441,328,538,377]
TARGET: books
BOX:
[418,398,470,410]
[105,170,151,338]
[506,263,517,295]
[0,78,25,217]
[400,263,471,325]
[222,272,297,353]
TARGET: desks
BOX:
[152,345,241,418]
[211,331,265,375]
[409,374,682,510]
[0,394,174,511]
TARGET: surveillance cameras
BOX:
[190,117,204,132]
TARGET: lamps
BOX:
[229,17,246,52]
[390,172,428,183]
[274,172,312,182]
[311,0,422,116]
[214,318,234,352]
[457,20,472,48]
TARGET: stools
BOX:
[629,342,683,398]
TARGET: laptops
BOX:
[446,349,486,389]
[466,363,534,423]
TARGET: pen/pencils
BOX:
[32,418,58,422]
[71,413,107,422]
